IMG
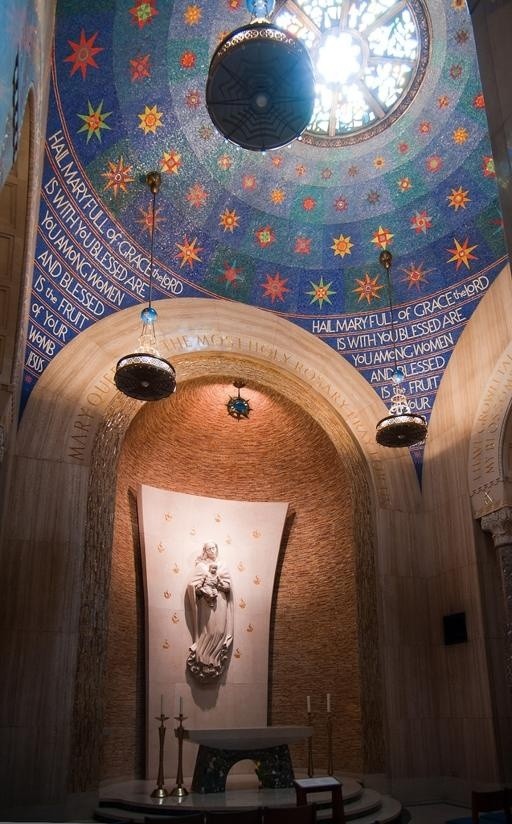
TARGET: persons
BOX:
[186,539,236,687]
[196,561,226,607]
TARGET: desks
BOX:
[172,724,315,793]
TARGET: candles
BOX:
[179,695,183,714]
[160,693,164,714]
[306,695,310,712]
[326,692,331,712]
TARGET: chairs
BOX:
[442,788,511,823]
[143,801,318,823]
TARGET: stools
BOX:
[290,775,344,824]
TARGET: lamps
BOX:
[372,236,427,447]
[115,169,177,402]
[202,1,315,154]
